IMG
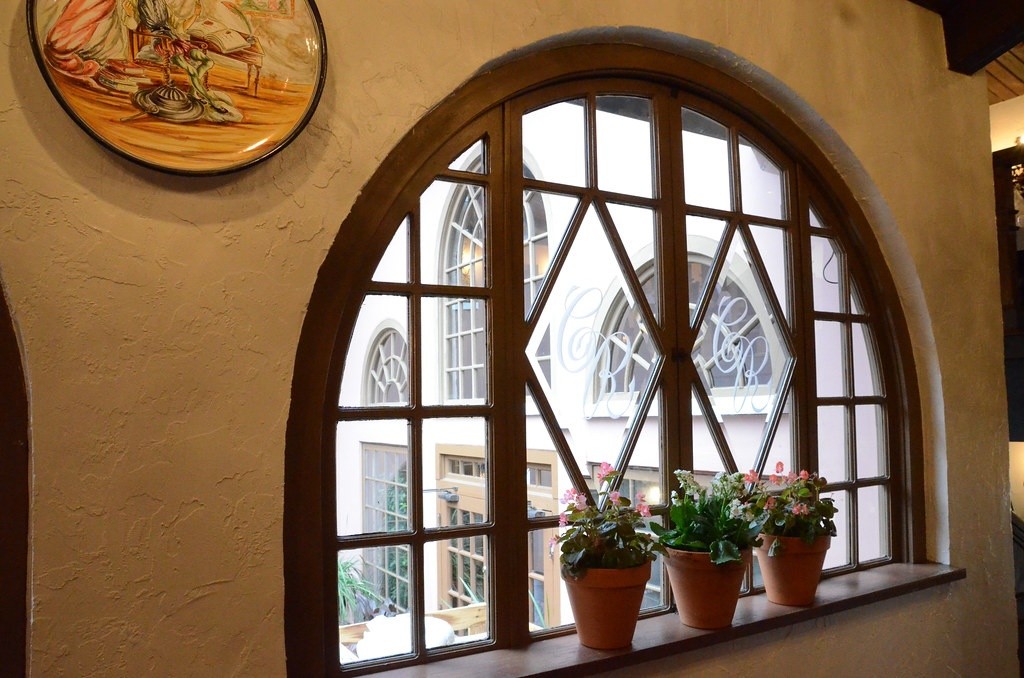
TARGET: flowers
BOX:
[548,463,670,581]
[649,470,772,565]
[754,463,840,556]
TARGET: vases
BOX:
[756,533,831,606]
[556,562,651,651]
[661,547,748,629]
